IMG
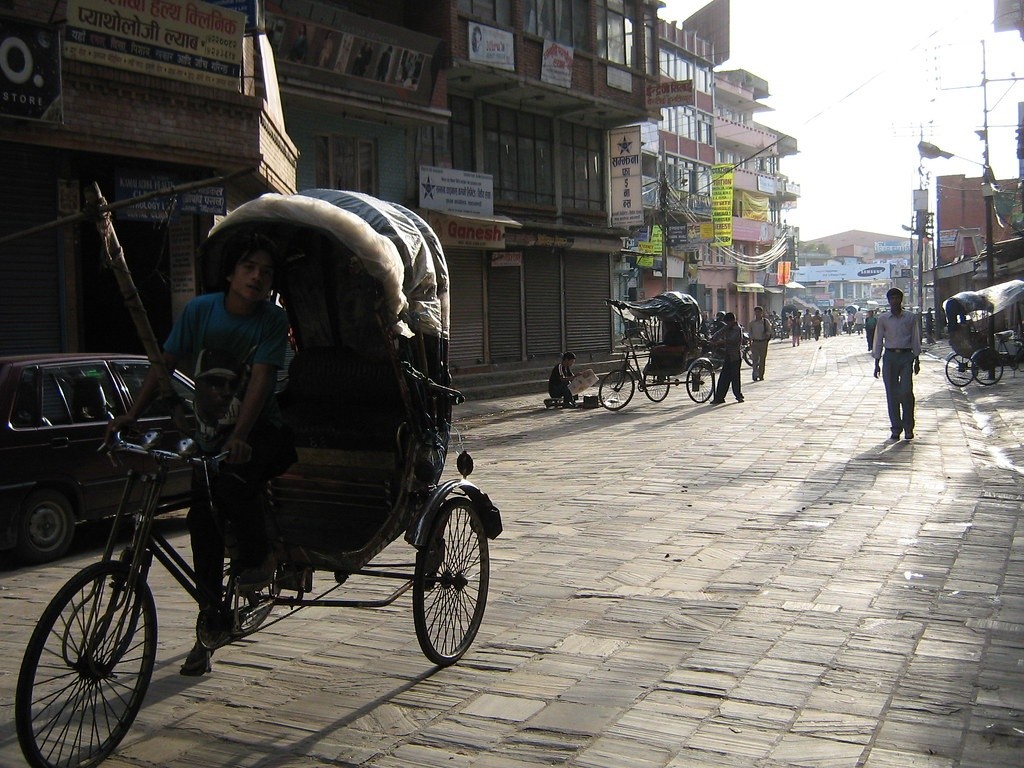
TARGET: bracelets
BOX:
[915,361,920,364]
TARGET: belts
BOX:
[885,347,911,353]
[754,339,765,342]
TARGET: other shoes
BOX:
[180,642,214,676]
[904,426,914,439]
[737,397,744,403]
[760,377,764,381]
[891,432,900,440]
[239,546,278,592]
[754,378,757,381]
[710,398,725,403]
[562,403,575,409]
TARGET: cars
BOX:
[0,352,204,565]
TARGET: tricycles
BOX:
[945,278,1024,386]
[14,186,502,768]
[598,290,717,413]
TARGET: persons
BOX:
[105,232,299,676]
[925,308,933,344]
[709,312,745,404]
[767,305,863,347]
[701,311,709,337]
[864,309,877,351]
[871,288,922,440]
[548,351,578,408]
[746,306,772,381]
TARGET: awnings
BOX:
[433,209,523,230]
[785,282,805,289]
[765,286,783,293]
[729,281,764,293]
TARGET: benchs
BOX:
[951,322,988,358]
[652,330,686,360]
[270,390,412,457]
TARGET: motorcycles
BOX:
[698,324,761,372]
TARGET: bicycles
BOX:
[969,318,1024,387]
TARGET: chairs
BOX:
[77,377,114,421]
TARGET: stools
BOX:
[544,398,564,409]
